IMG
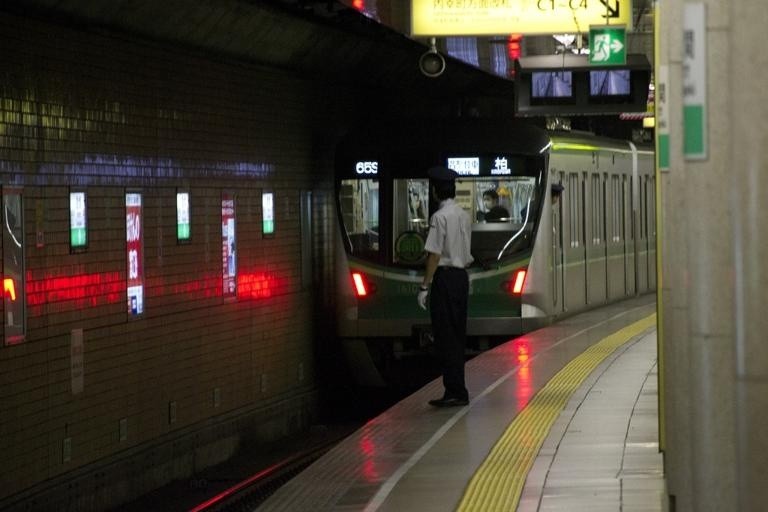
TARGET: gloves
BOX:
[419,285,427,310]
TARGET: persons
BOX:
[408,192,425,218]
[482,188,512,223]
[416,165,476,408]
[520,182,566,223]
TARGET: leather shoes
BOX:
[428,392,470,408]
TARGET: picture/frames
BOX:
[125,187,145,323]
[69,186,89,255]
[261,190,276,237]
[176,188,193,246]
[0,185,27,347]
[221,191,239,305]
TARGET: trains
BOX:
[321,105,659,416]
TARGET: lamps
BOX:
[419,37,446,77]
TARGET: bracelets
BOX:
[419,286,429,291]
